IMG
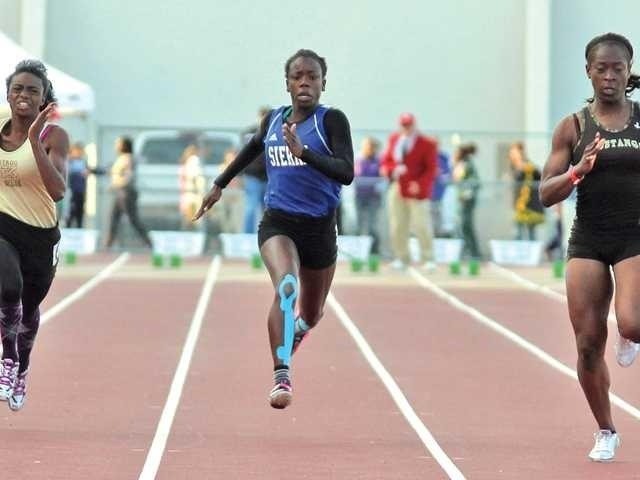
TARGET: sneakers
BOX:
[290,315,308,356]
[269,383,293,410]
[615,327,639,368]
[0,353,19,400]
[421,261,437,273]
[589,430,620,462]
[389,258,408,270]
[8,368,30,411]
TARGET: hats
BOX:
[399,111,415,126]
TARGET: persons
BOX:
[0,59,69,412]
[537,30,639,463]
[185,45,358,409]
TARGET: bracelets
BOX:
[568,166,586,187]
